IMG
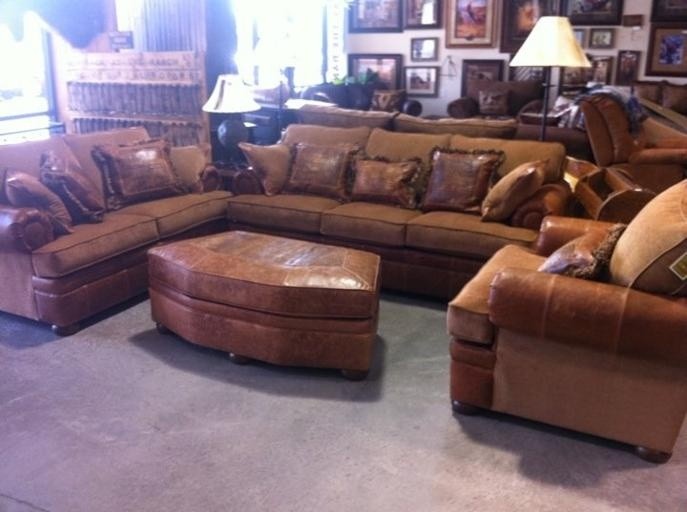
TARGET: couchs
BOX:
[0,126,236,337]
[300,105,399,127]
[228,124,571,299]
[515,122,596,164]
[301,83,422,116]
[147,231,380,381]
[394,113,519,137]
[579,96,687,193]
[447,80,544,123]
[448,178,687,464]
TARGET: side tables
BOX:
[214,162,252,195]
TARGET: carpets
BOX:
[0,299,687,512]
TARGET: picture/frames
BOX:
[499,0,562,53]
[615,50,640,85]
[650,0,687,22]
[508,53,548,83]
[411,38,438,61]
[644,21,687,77]
[403,66,440,97]
[573,29,585,49]
[562,0,623,26]
[347,0,403,33]
[445,0,499,49]
[403,0,442,29]
[461,59,503,96]
[348,54,402,89]
[592,56,613,85]
[589,28,614,48]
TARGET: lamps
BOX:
[509,16,592,141]
[202,74,261,172]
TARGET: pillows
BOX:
[350,155,425,208]
[610,179,687,295]
[660,80,687,110]
[5,169,75,234]
[419,145,506,214]
[481,158,551,221]
[631,80,663,105]
[39,137,105,223]
[281,142,361,204]
[537,224,625,277]
[238,138,302,196]
[170,142,211,195]
[478,88,510,114]
[368,88,406,112]
[327,83,348,106]
[92,137,191,210]
[520,113,563,126]
[347,81,366,107]
